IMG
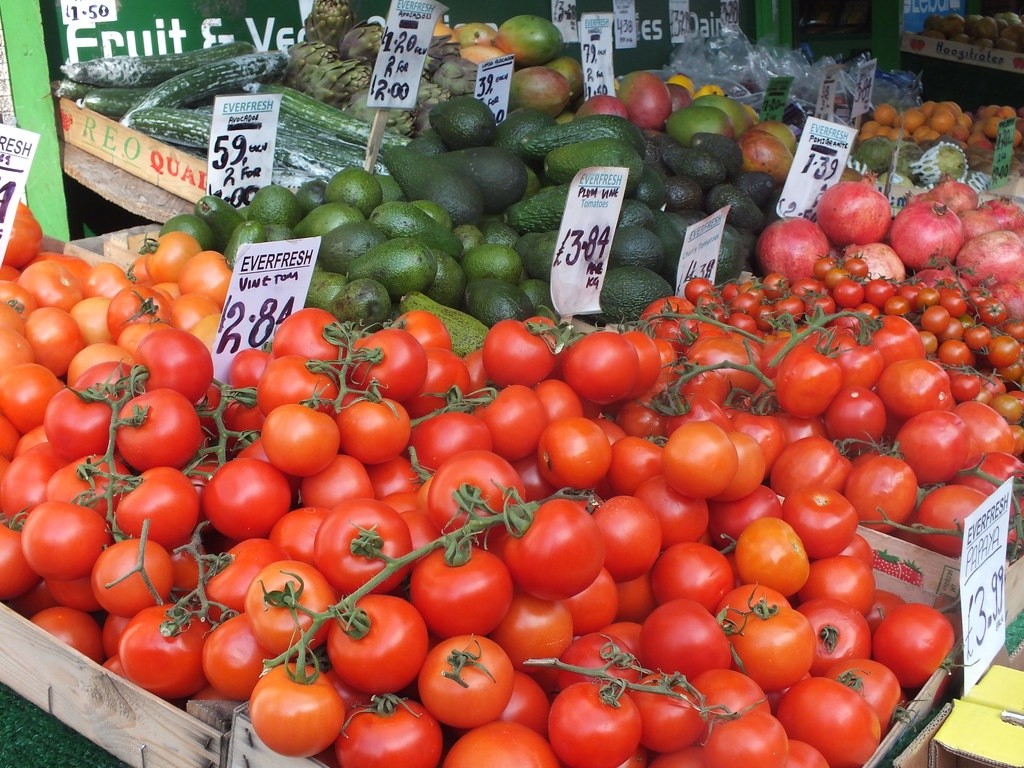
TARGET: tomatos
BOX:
[1,202,1024,768]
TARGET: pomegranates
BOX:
[756,171,1024,317]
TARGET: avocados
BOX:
[159,98,779,358]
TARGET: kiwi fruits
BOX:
[915,135,1024,181]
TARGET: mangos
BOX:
[430,14,795,178]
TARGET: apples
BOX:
[1013,57,1024,69]
[919,10,1024,54]
[911,39,925,50]
